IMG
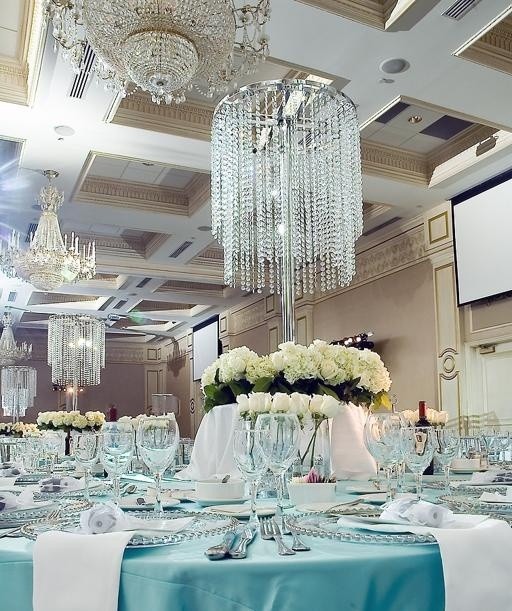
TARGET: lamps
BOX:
[0,314,33,367]
[1,366,37,417]
[212,79,363,296]
[40,0,271,107]
[47,314,105,387]
[0,184,96,291]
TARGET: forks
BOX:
[0,509,62,538]
[258,517,295,555]
[280,513,310,549]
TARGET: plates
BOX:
[17,495,90,509]
[110,496,179,509]
[34,483,113,495]
[347,483,406,491]
[284,507,512,542]
[296,502,376,512]
[186,494,249,506]
[360,493,428,501]
[436,492,511,509]
[20,510,239,546]
[204,504,277,515]
[444,479,510,493]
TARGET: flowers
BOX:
[0,421,39,438]
[201,339,392,414]
[236,392,340,470]
[35,410,105,455]
[401,408,448,453]
[118,414,170,447]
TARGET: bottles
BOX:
[292,457,302,478]
[314,454,323,477]
[480,449,488,468]
[472,452,481,459]
[413,400,434,475]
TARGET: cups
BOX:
[400,428,437,516]
[363,413,411,511]
[482,429,494,465]
[197,478,243,499]
[460,438,475,458]
[137,418,179,516]
[257,415,298,525]
[494,432,510,463]
[430,430,460,500]
[231,421,272,532]
[73,434,103,505]
[100,434,133,508]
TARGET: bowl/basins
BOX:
[287,483,337,504]
